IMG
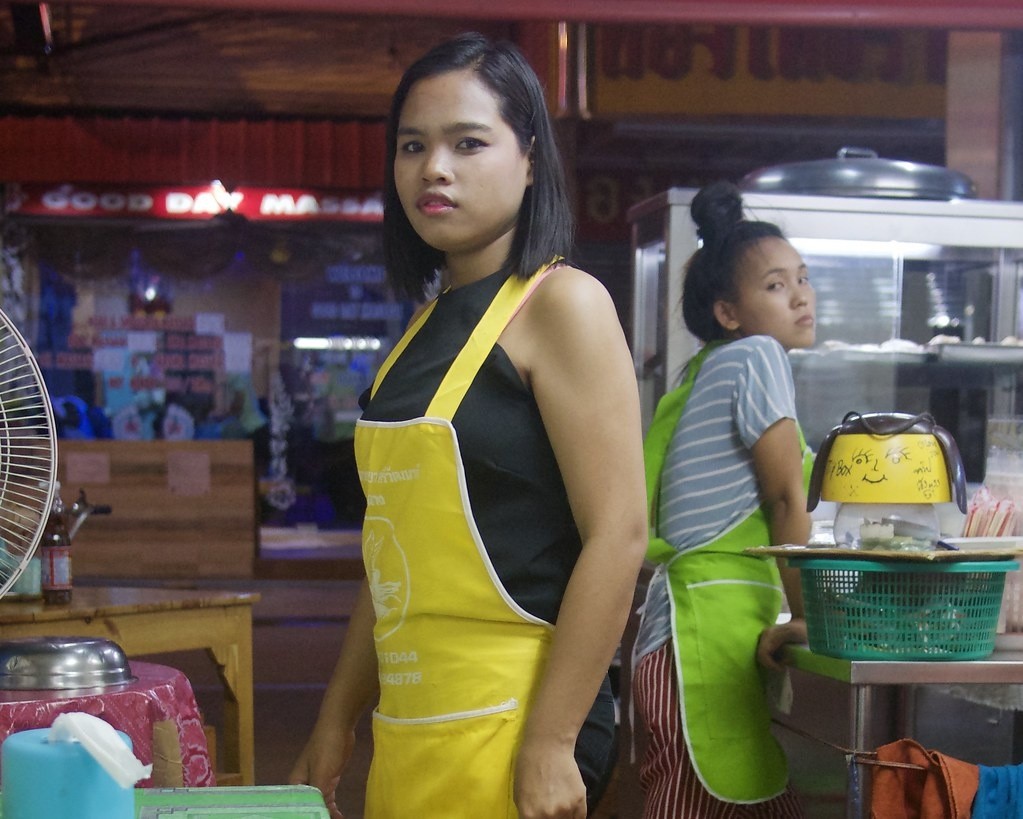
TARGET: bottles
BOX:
[39,482,72,602]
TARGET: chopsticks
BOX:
[963,486,1017,537]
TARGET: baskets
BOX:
[784,557,1022,663]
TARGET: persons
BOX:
[291,31,650,819]
[631,182,817,819]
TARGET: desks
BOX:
[134,784,333,819]
[0,587,262,787]
[771,641,1023,819]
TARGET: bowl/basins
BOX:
[0,636,139,691]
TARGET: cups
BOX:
[8,557,41,593]
